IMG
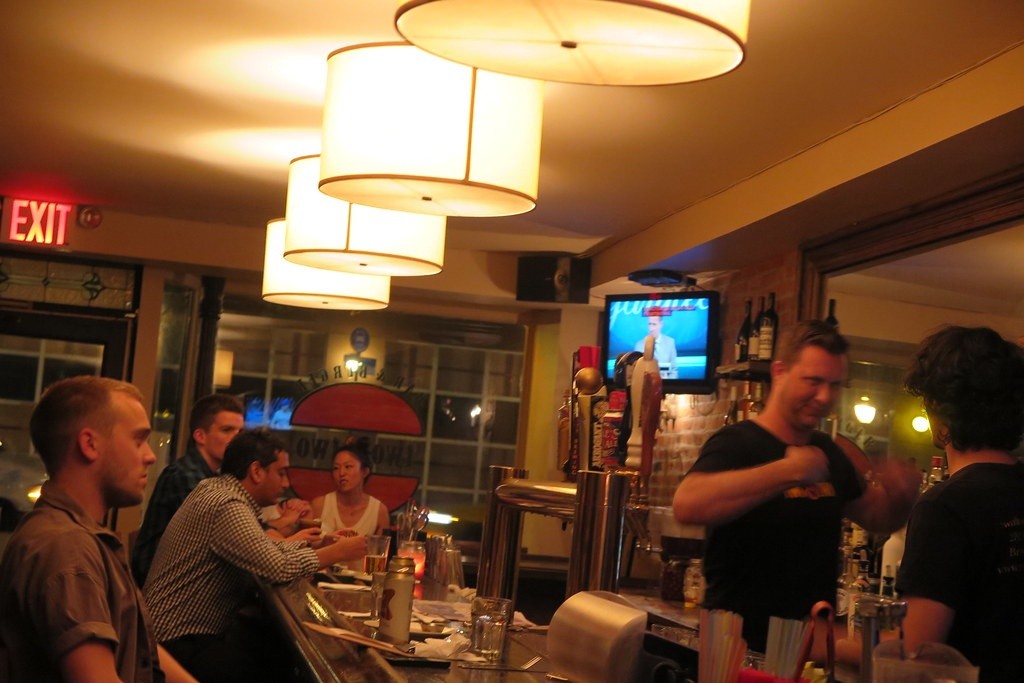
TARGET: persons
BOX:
[311,435,389,547]
[810,325,1024,683]
[0,375,201,683]
[143,430,368,683]
[132,393,313,604]
[673,320,923,655]
[636,306,678,379]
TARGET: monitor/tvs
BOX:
[604,290,721,395]
[243,395,294,430]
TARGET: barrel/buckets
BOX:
[380,529,426,571]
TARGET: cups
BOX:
[472,596,512,664]
[430,534,466,590]
[371,572,388,619]
[368,536,391,572]
[364,556,387,573]
[396,531,417,547]
[652,624,767,673]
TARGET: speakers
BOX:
[516,255,591,304]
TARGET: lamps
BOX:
[282,152,446,276]
[79,207,103,229]
[394,0,750,86]
[260,215,390,310]
[319,36,542,216]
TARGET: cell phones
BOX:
[384,656,452,669]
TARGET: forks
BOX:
[462,656,542,670]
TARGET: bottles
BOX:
[398,499,429,531]
[735,292,778,361]
[918,455,949,496]
[835,532,895,640]
[725,380,764,427]
[662,556,706,605]
[825,299,839,334]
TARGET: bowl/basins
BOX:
[333,570,373,586]
[421,622,445,633]
[364,620,457,644]
